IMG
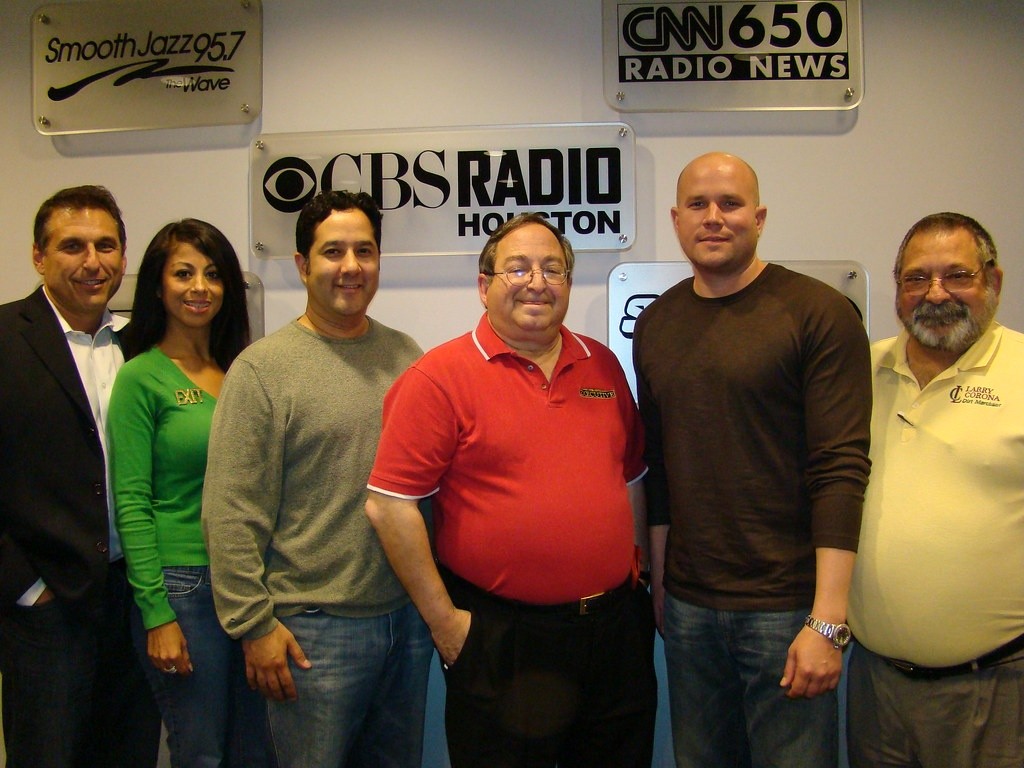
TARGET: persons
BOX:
[204,191,435,768]
[843,211,1024,768]
[635,151,873,768]
[366,215,660,767]
[104,218,252,768]
[0,185,149,768]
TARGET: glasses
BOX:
[494,264,571,287]
[896,265,983,297]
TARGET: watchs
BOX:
[805,615,851,649]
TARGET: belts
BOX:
[845,620,1024,680]
[460,573,631,617]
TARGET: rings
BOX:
[164,666,177,674]
